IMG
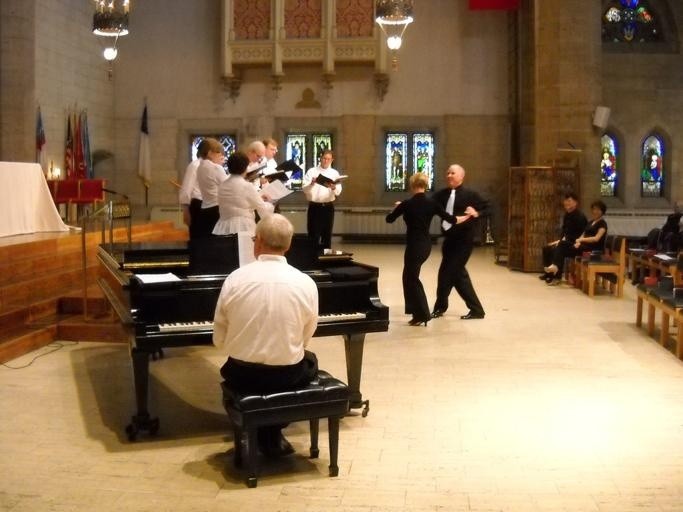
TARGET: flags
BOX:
[34,106,48,182]
[136,96,152,190]
[71,101,85,181]
[81,107,92,180]
[64,106,72,182]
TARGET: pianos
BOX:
[96,232,389,443]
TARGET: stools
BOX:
[220,370,351,487]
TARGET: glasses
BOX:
[271,148,278,151]
[252,236,258,240]
[322,157,335,161]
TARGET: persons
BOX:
[238,139,265,189]
[632,200,682,288]
[385,173,472,329]
[260,137,282,214]
[196,135,229,238]
[544,201,607,286]
[426,164,494,321]
[302,150,341,249]
[212,213,319,461]
[539,193,587,282]
[209,152,275,240]
[178,144,224,241]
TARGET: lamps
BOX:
[90,0,132,82]
[372,0,415,72]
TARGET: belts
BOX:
[229,350,310,370]
[310,199,335,206]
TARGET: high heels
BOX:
[544,264,559,275]
[409,315,430,327]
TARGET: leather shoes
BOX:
[546,275,553,282]
[270,433,296,459]
[462,312,484,319]
[431,308,444,317]
[256,442,265,466]
[540,273,546,279]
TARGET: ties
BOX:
[442,190,456,230]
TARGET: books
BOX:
[315,173,349,189]
[265,172,291,183]
[275,159,304,177]
[244,162,267,180]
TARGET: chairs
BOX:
[636,264,683,333]
[647,288,683,346]
[582,236,626,297]
[565,257,568,280]
[661,295,683,359]
[625,228,683,285]
[45,180,57,202]
[568,256,582,285]
[577,235,614,290]
[56,180,80,223]
[71,180,106,226]
